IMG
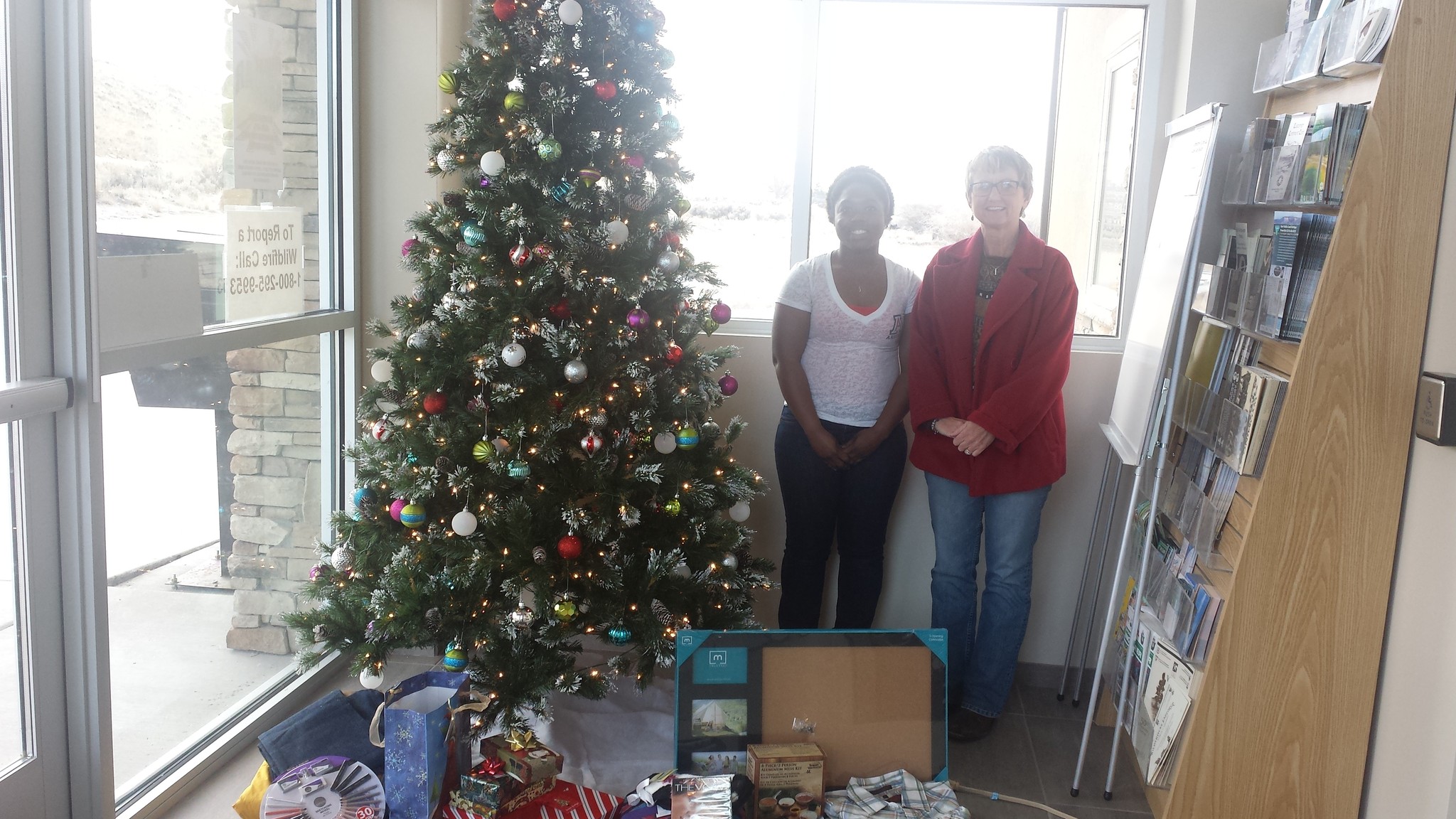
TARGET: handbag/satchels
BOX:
[369,671,491,819]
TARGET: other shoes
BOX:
[948,707,995,742]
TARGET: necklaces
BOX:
[983,247,1011,275]
[838,251,875,292]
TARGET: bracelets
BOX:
[931,418,939,435]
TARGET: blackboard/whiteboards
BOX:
[1097,103,1229,465]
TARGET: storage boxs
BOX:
[440,726,625,818]
[747,740,827,819]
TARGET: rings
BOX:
[966,448,973,455]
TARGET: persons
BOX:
[907,145,1078,741]
[771,167,923,629]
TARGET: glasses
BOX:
[969,180,1023,197]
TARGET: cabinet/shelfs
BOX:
[1094,1,1456,819]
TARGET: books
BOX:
[1109,0,1402,785]
